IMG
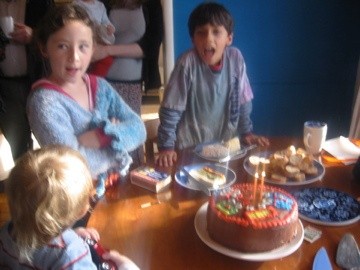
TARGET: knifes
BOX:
[312,246,332,270]
[219,144,256,162]
[177,165,211,197]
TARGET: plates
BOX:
[175,164,237,191]
[289,187,360,226]
[243,152,326,186]
[194,200,305,261]
[193,141,247,162]
[97,23,115,44]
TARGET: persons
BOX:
[104,0,164,166]
[27,1,146,202]
[68,1,115,78]
[154,3,270,167]
[0,144,139,270]
[0,0,52,160]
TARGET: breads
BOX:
[188,168,226,191]
[201,136,240,157]
[248,145,318,184]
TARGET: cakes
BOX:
[206,183,298,254]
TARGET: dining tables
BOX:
[87,137,360,270]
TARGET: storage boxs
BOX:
[130,166,172,193]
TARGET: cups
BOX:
[303,121,328,154]
[313,155,322,180]
[0,16,15,38]
